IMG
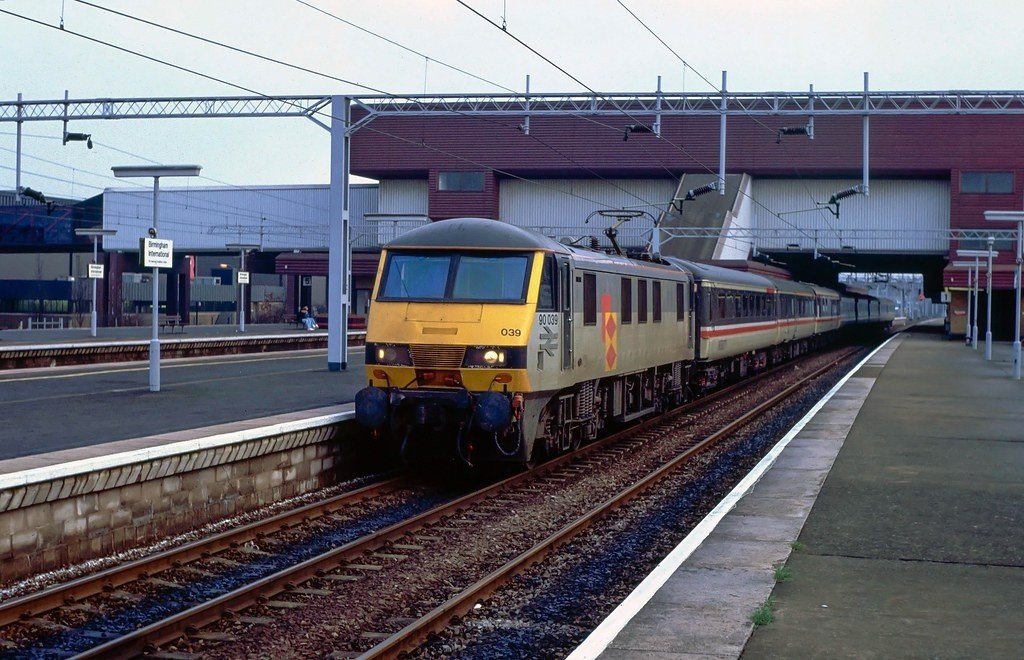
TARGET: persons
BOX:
[298,306,319,331]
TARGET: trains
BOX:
[354,217,899,474]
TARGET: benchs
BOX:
[158,316,188,334]
[282,314,305,329]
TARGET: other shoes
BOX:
[312,324,319,328]
[307,328,315,331]
